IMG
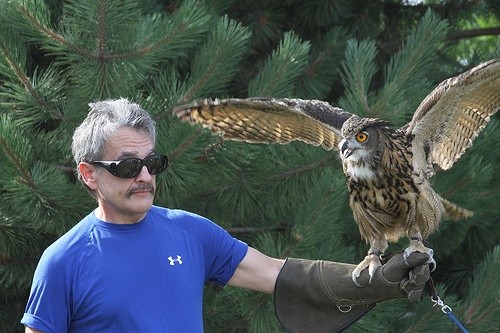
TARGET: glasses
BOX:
[80,154,169,179]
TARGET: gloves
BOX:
[274,247,432,333]
